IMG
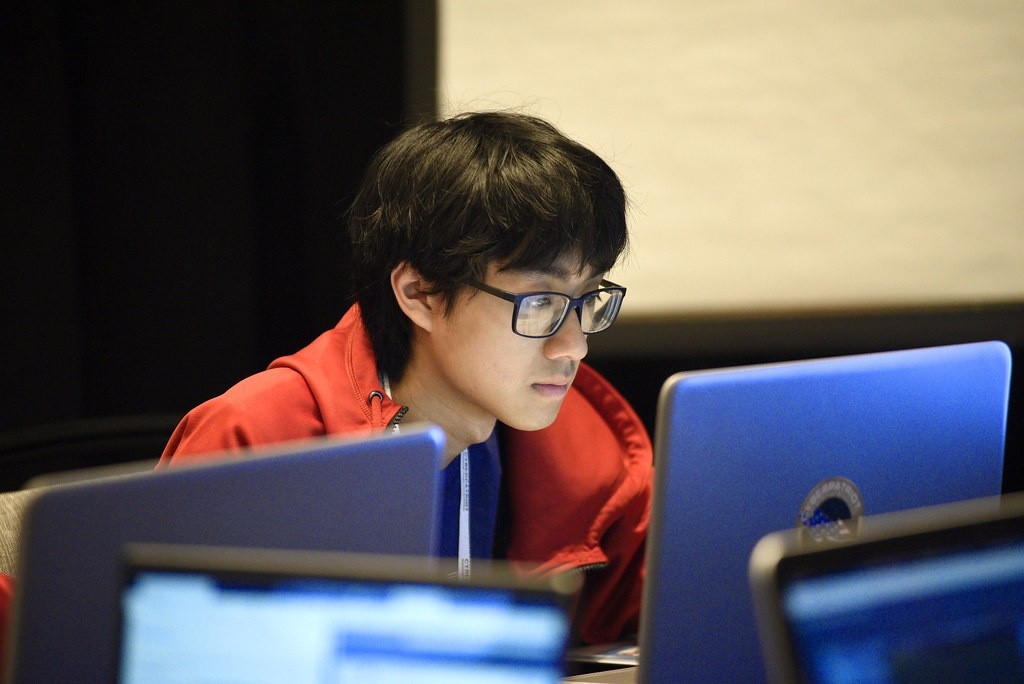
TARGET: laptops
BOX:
[563,340,1011,668]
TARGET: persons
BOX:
[149,109,658,651]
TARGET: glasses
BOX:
[453,274,626,336]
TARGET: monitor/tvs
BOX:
[115,539,577,683]
[747,489,1023,684]
[0,426,443,683]
